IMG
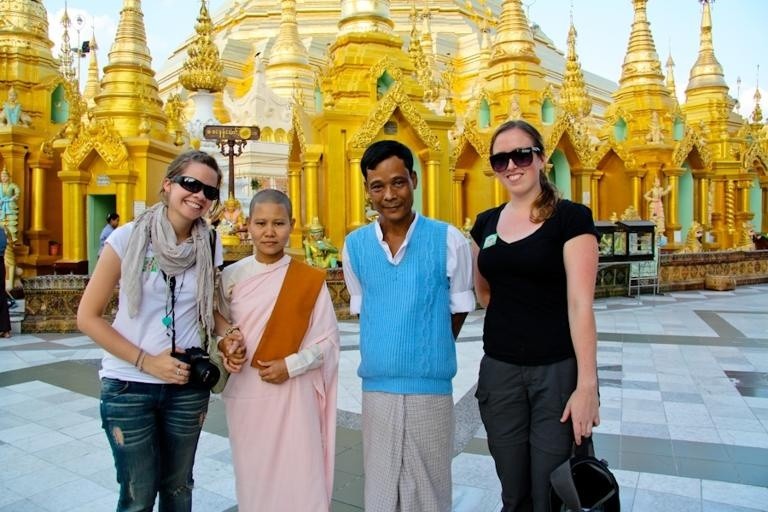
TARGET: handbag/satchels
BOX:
[548,436,621,512]
[198,329,230,394]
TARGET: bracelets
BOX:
[139,353,149,372]
[134,348,144,368]
[223,324,240,338]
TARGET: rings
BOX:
[177,369,182,374]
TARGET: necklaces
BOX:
[161,270,186,338]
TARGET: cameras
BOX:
[171,346,220,391]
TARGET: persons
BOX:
[0,83,33,128]
[0,164,21,246]
[217,191,248,238]
[643,170,672,246]
[0,225,12,339]
[96,212,119,261]
[303,217,339,274]
[466,120,602,512]
[213,186,341,512]
[75,149,248,512]
[339,139,480,512]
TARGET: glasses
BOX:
[169,176,219,200]
[490,146,541,172]
